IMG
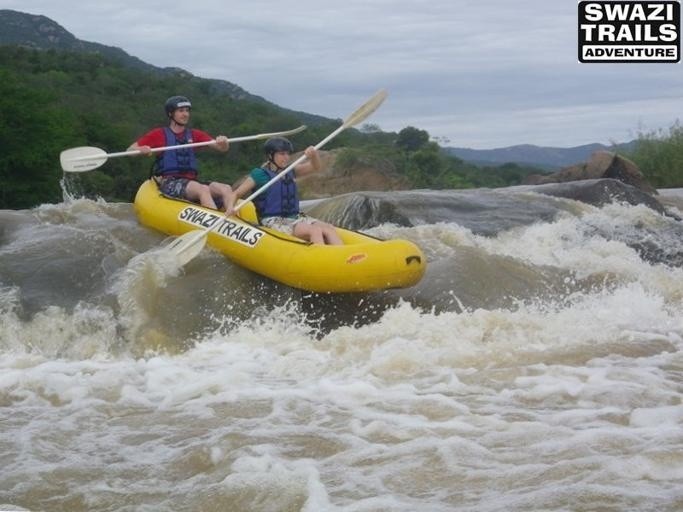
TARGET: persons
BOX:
[127,96,233,210]
[224,136,344,244]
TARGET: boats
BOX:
[134,174,426,295]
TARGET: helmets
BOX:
[163,94,193,116]
[263,136,296,156]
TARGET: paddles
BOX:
[168,91,387,266]
[59,126,309,173]
